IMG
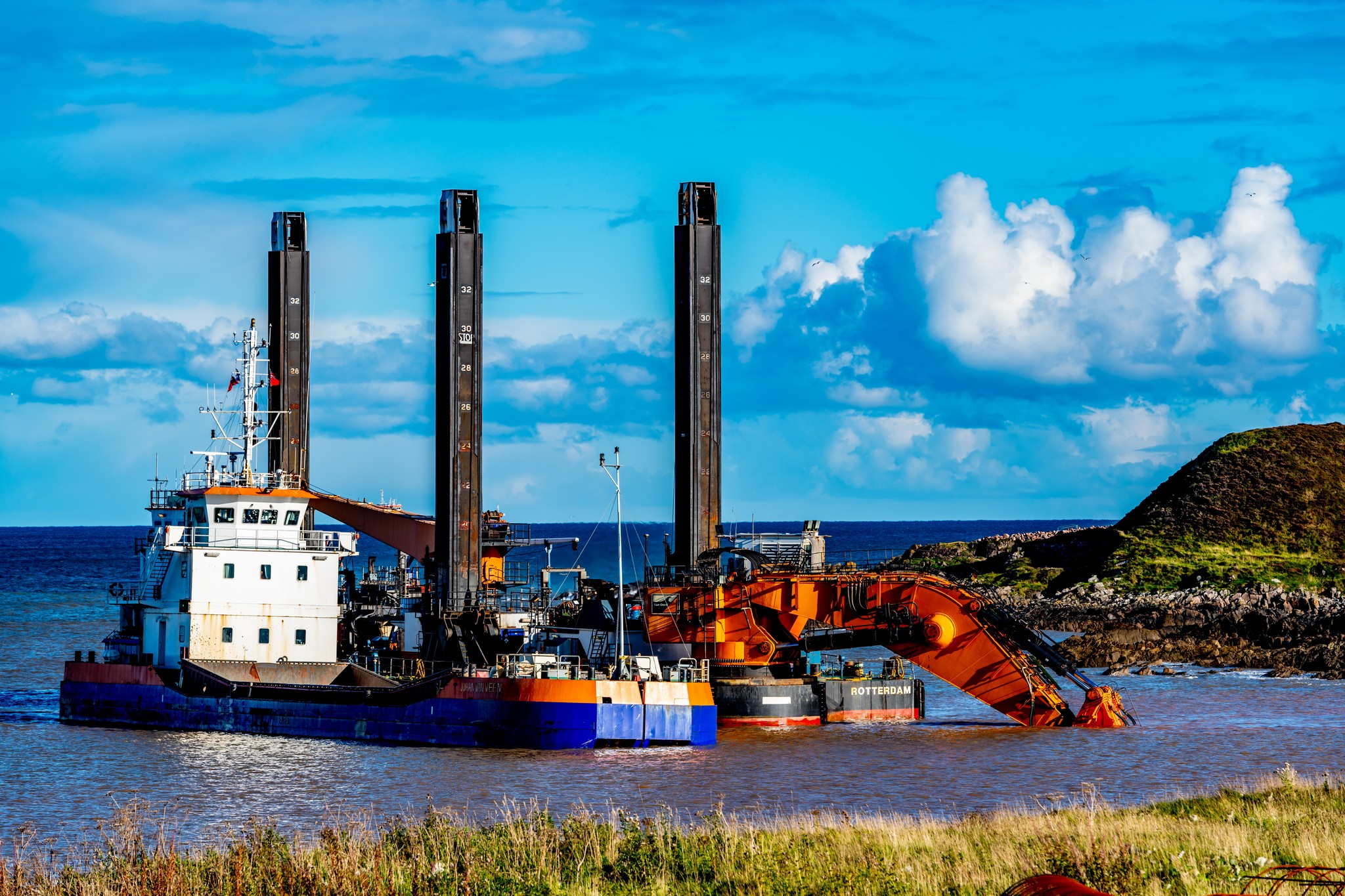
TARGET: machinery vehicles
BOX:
[637,518,1137,730]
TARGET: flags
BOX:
[235,368,241,386]
[227,376,238,392]
[270,369,280,387]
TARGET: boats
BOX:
[58,315,722,752]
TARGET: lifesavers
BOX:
[147,529,155,544]
[110,583,123,596]
